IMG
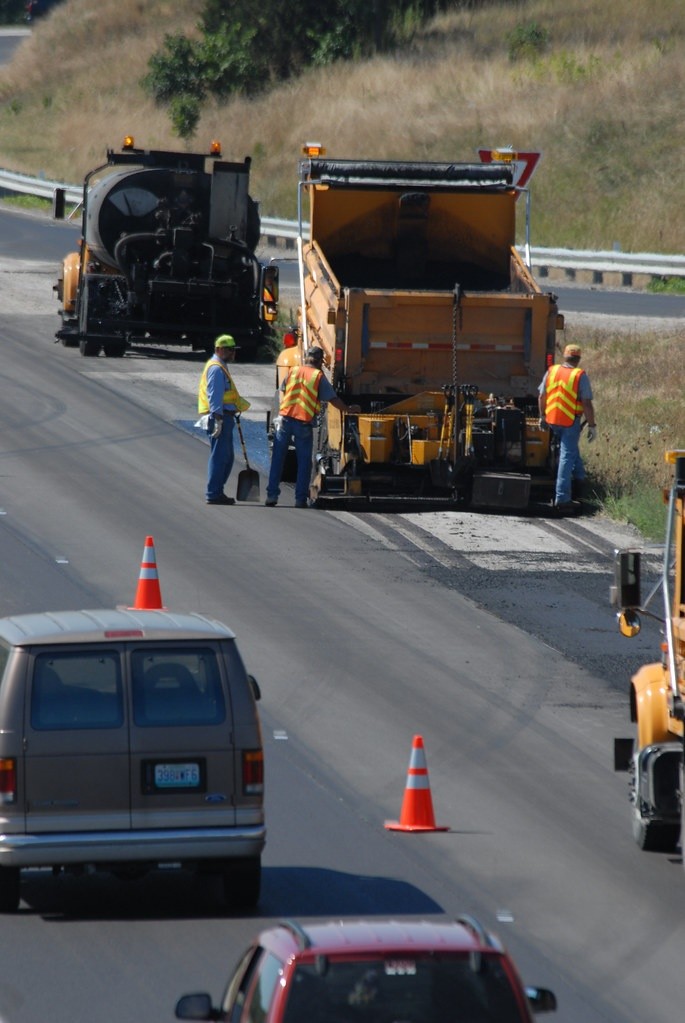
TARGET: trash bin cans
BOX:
[52,187,66,219]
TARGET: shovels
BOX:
[233,409,262,503]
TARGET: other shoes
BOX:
[265,499,278,507]
[555,499,580,509]
[206,493,235,505]
[296,501,308,507]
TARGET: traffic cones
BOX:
[118,537,169,613]
[384,735,451,832]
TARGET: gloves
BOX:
[539,415,548,432]
[211,420,224,438]
[587,423,597,442]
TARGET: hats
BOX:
[215,335,240,349]
[564,344,581,356]
[308,346,325,363]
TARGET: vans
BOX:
[1,604,267,915]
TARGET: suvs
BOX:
[175,914,560,1022]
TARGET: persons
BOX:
[264,347,361,509]
[537,345,598,512]
[197,334,252,506]
[347,970,384,1005]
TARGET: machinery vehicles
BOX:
[610,447,684,862]
[260,143,565,511]
[53,134,269,362]
